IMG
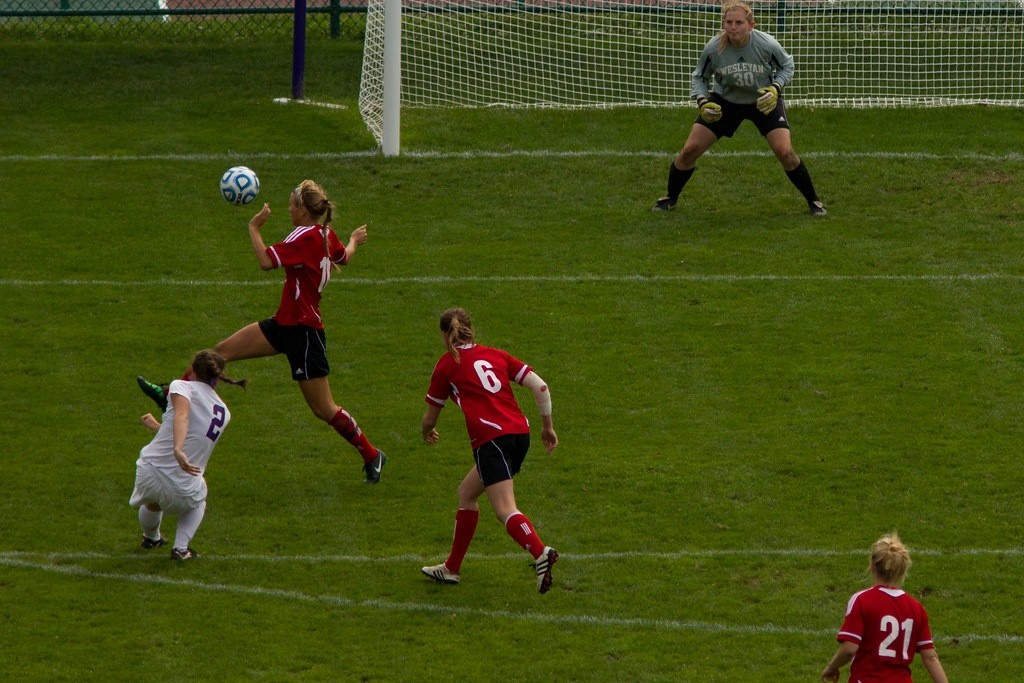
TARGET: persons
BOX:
[820,530,951,683]
[420,306,559,594]
[128,348,249,564]
[652,1,827,218]
[136,180,389,484]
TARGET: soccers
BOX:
[219,165,260,207]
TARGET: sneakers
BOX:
[362,449,388,484]
[141,533,168,549]
[652,195,678,213]
[809,201,827,217]
[170,548,201,562]
[137,376,171,412]
[529,545,558,594]
[421,560,460,584]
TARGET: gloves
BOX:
[756,85,779,116]
[700,102,722,124]
